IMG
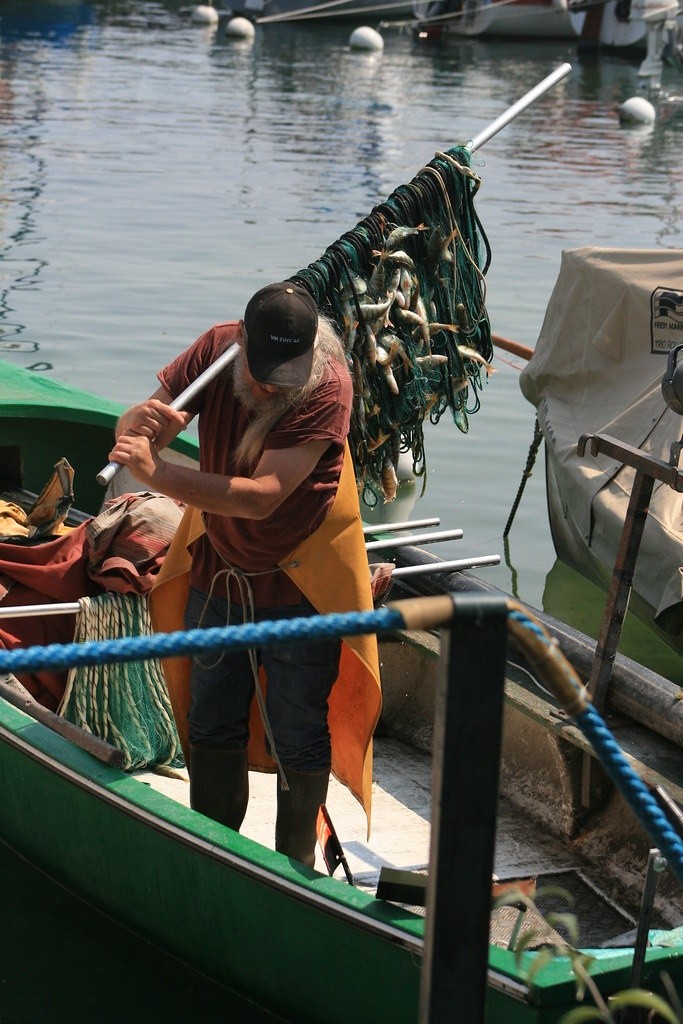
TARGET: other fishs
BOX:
[357,427,392,457]
[452,370,468,396]
[457,302,470,332]
[381,455,401,505]
[417,392,438,421]
[456,345,496,379]
[338,222,460,402]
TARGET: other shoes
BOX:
[276,765,331,869]
[188,740,250,834]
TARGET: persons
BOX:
[108,282,376,869]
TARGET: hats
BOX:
[244,281,319,388]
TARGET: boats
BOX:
[0,354,683,1022]
[520,242,683,669]
